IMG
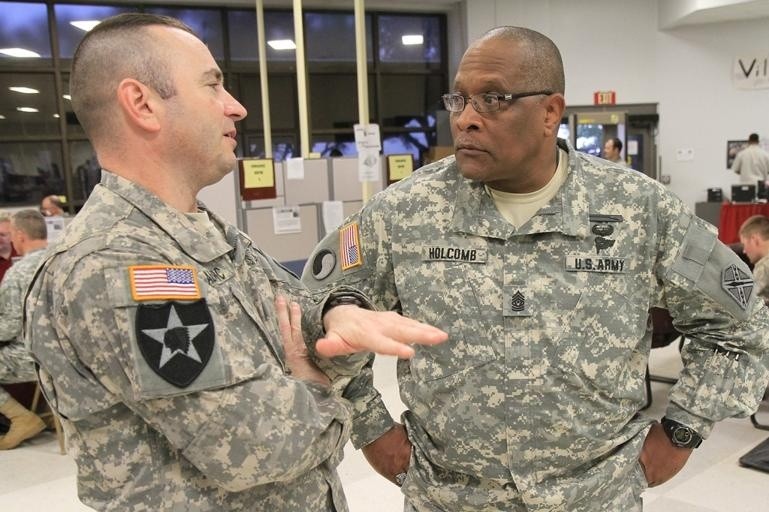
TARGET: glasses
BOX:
[442,90,553,113]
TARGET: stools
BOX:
[25,366,59,442]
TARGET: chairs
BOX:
[639,304,691,409]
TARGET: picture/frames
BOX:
[726,140,751,170]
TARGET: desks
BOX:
[720,201,769,246]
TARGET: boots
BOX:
[0,398,46,450]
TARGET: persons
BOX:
[734,214,769,309]
[298,21,769,512]
[731,130,769,195]
[20,11,452,511]
[601,138,629,168]
[0,195,74,451]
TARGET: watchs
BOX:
[660,415,703,451]
